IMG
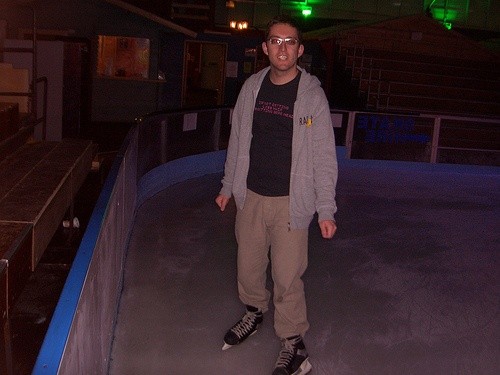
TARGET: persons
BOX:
[216,14,338,375]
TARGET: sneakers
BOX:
[224,305,264,345]
[269,334,312,375]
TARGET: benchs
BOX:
[338,43,500,120]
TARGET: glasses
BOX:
[267,36,301,45]
